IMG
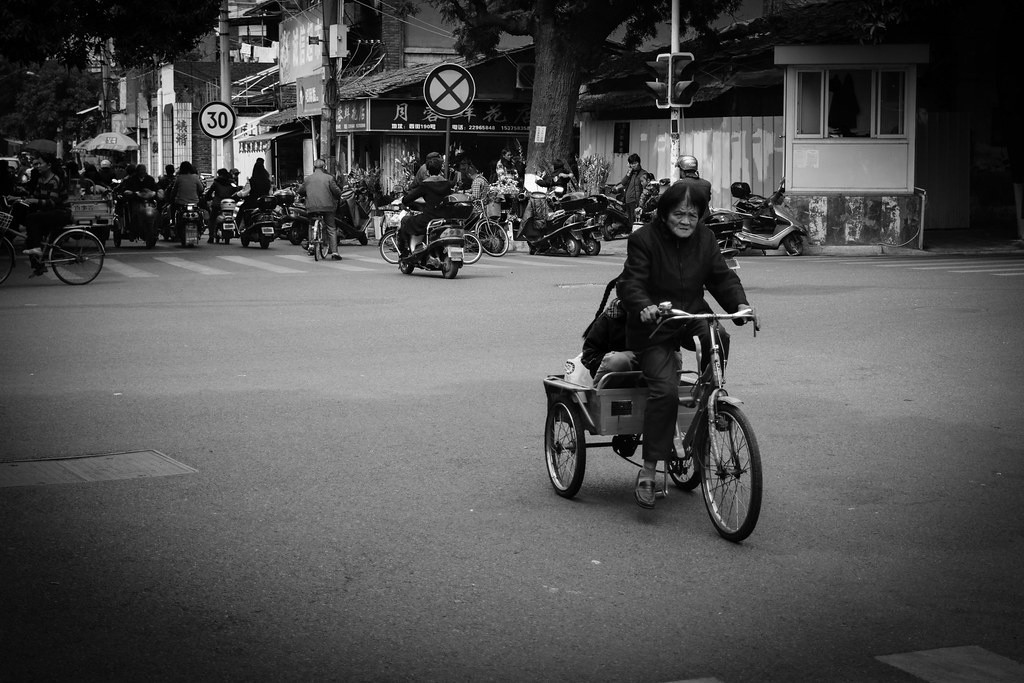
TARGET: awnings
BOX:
[239,129,296,152]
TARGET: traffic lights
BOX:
[669,53,700,108]
[645,53,671,109]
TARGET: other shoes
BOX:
[22,247,42,256]
[308,246,315,255]
[331,253,342,260]
[27,267,48,279]
[399,247,410,258]
[207,238,214,243]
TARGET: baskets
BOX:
[486,204,501,218]
[0,211,13,234]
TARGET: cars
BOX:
[0,157,22,171]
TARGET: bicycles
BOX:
[378,191,509,265]
[0,194,106,286]
[311,213,330,262]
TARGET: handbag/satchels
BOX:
[564,352,597,403]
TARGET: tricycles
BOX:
[544,301,763,543]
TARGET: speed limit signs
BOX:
[198,101,236,139]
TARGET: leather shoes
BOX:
[716,414,729,431]
[634,469,656,509]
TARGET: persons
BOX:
[298,159,342,259]
[0,155,271,243]
[583,178,761,509]
[403,147,580,237]
[398,159,452,258]
[669,155,713,226]
[621,155,660,236]
[6,156,67,278]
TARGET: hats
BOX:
[230,168,241,174]
[427,152,444,162]
[314,159,325,168]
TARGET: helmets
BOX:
[101,160,111,169]
[678,155,698,171]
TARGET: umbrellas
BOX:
[70,138,102,154]
[85,132,140,154]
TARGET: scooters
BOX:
[398,193,475,280]
[110,180,374,250]
[703,177,808,259]
[67,177,117,253]
[516,177,671,258]
